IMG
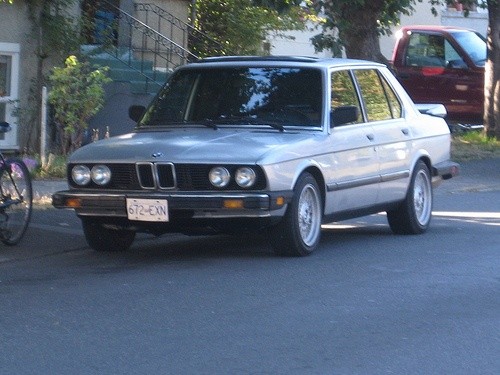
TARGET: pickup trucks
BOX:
[388,25,487,122]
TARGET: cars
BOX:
[52,54,459,257]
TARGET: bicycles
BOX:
[0,122,33,246]
